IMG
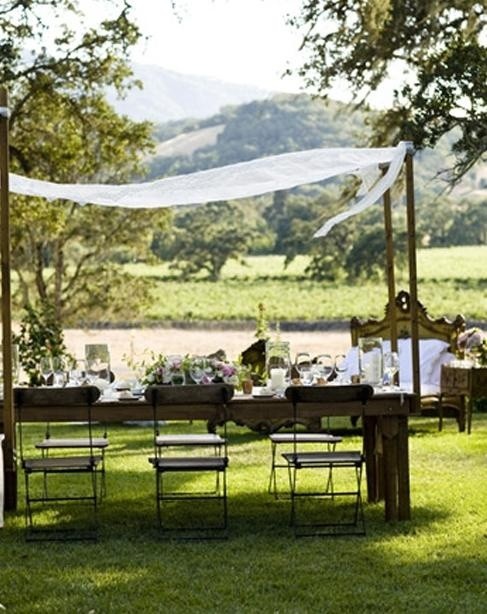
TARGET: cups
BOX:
[242,379,254,395]
[358,336,385,392]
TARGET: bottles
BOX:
[266,340,292,399]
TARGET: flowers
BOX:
[457,328,487,358]
[130,338,248,387]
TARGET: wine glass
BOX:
[189,358,218,385]
[382,351,401,392]
[39,355,111,388]
[295,353,348,386]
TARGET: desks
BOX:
[439,363,487,435]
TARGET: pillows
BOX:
[341,339,453,392]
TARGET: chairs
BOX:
[16,385,372,544]
